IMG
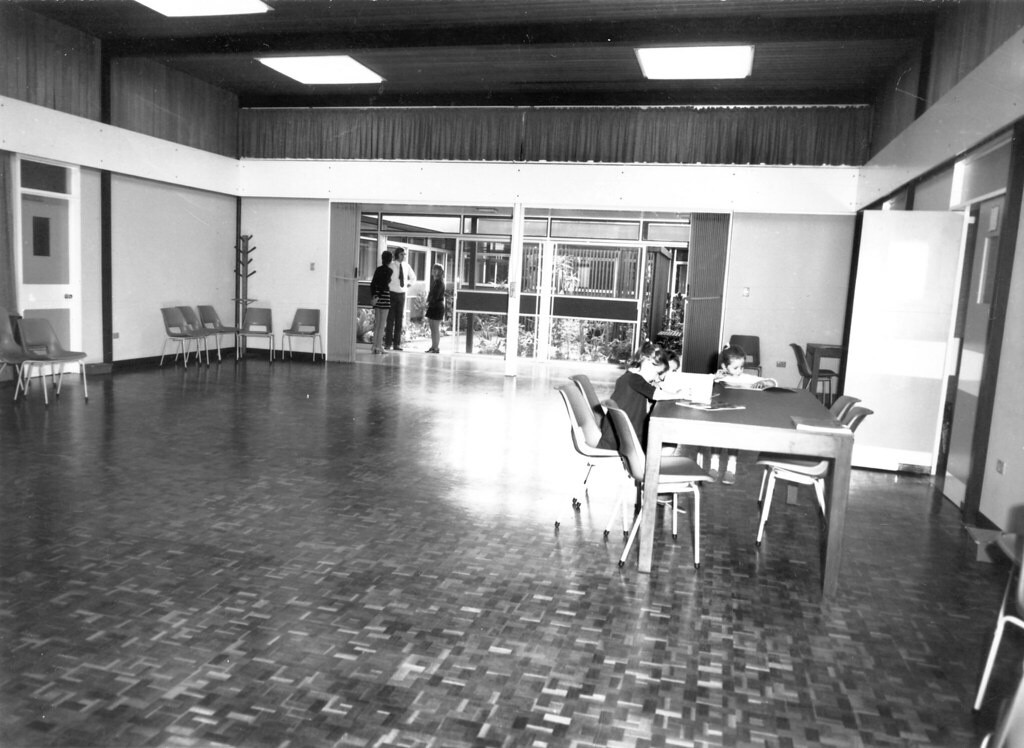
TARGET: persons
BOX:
[709,345,780,485]
[426,263,445,353]
[602,339,692,514]
[385,248,417,351]
[372,251,393,354]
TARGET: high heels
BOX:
[372,346,389,354]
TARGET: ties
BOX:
[399,263,404,287]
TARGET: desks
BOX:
[657,330,683,347]
[637,382,855,597]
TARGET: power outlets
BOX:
[113,332,119,338]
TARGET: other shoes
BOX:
[394,345,403,351]
[706,469,719,482]
[656,493,684,513]
[384,344,390,349]
[426,348,439,353]
[722,470,736,485]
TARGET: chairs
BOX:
[0,306,89,408]
[159,305,325,370]
[552,334,876,569]
